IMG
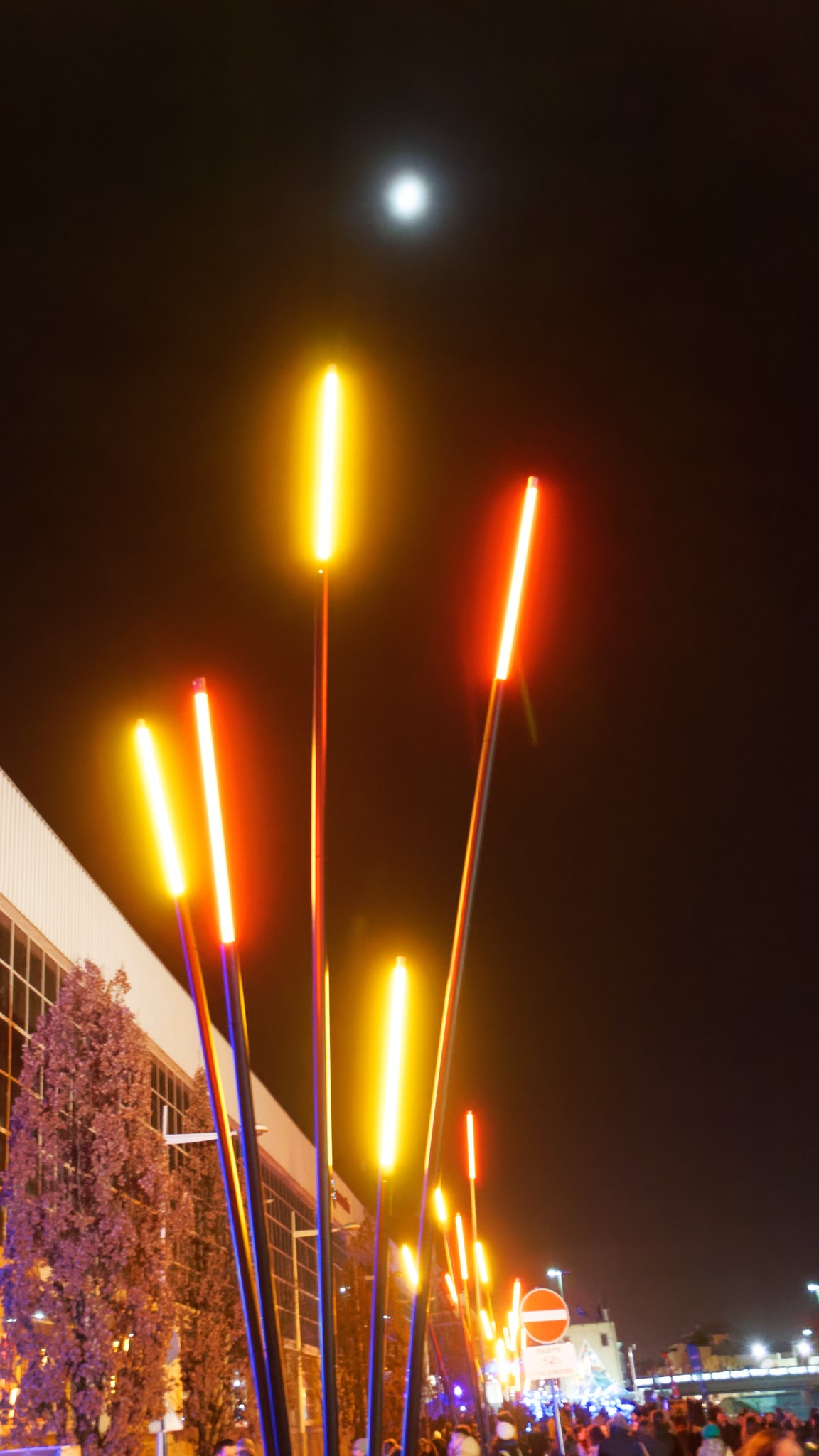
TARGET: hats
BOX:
[237,1438,255,1448]
[354,1437,368,1453]
[610,1414,630,1431]
[703,1424,720,1438]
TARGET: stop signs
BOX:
[520,1288,571,1345]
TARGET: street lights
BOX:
[155,1105,269,1456]
[546,1267,567,1298]
[288,1210,363,1455]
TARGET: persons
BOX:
[212,1396,819,1456]
